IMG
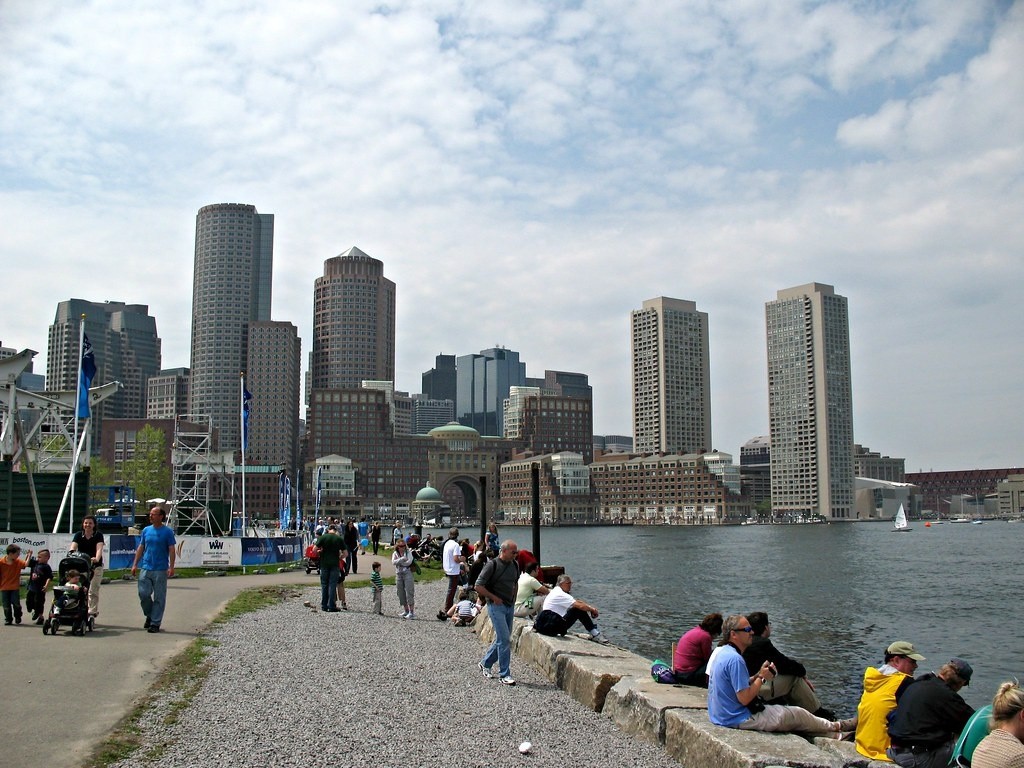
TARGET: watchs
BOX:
[756,676,767,686]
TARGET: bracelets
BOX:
[588,605,590,610]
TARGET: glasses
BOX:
[734,627,753,632]
[767,621,772,626]
[512,551,519,555]
[150,513,161,516]
[398,545,407,548]
[566,582,572,585]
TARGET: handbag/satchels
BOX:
[410,560,416,572]
[533,609,567,637]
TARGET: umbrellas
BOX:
[146,497,166,506]
[115,498,141,506]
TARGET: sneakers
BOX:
[478,662,493,678]
[590,632,609,644]
[498,675,516,685]
[403,612,417,619]
[398,612,409,617]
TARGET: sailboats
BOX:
[891,502,913,532]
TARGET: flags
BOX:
[74,330,98,418]
[243,386,250,453]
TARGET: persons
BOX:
[250,513,358,612]
[131,508,176,635]
[707,614,859,742]
[357,518,370,555]
[0,544,33,625]
[544,572,609,643]
[511,562,550,619]
[25,548,53,626]
[674,613,725,690]
[947,673,1024,768]
[390,520,403,545]
[854,641,927,763]
[392,538,416,620]
[742,611,838,721]
[71,512,105,618]
[371,521,381,555]
[886,656,976,768]
[368,562,385,616]
[474,540,521,688]
[408,526,501,628]
[59,566,87,607]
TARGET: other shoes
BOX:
[437,609,447,621]
[32,612,39,620]
[4,621,12,626]
[35,616,44,624]
[144,617,151,628]
[454,618,462,626]
[839,731,855,741]
[373,610,384,615]
[147,625,160,633]
[322,604,347,613]
[839,716,858,731]
[14,617,21,624]
[88,615,94,621]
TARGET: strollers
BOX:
[42,548,98,637]
[304,542,322,575]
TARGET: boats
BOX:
[929,494,944,524]
[950,493,971,523]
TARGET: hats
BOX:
[946,656,974,687]
[887,641,927,661]
